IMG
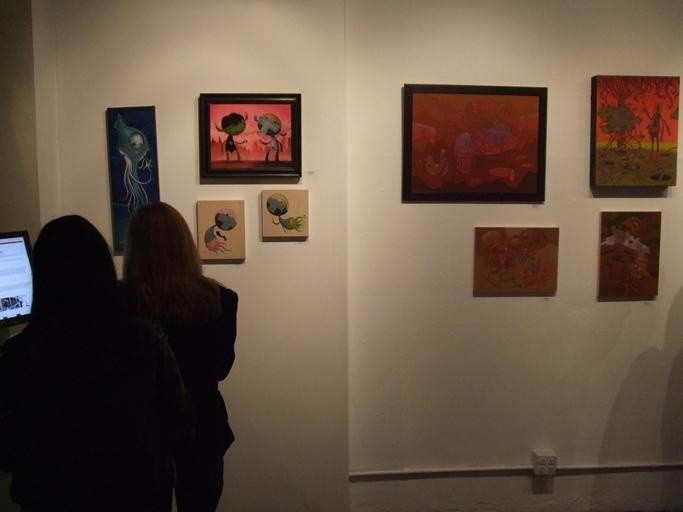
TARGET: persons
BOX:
[1,215,195,511]
[118,201,238,510]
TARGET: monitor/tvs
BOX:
[0,230,36,329]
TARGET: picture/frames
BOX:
[402,84,547,203]
[198,93,302,177]
